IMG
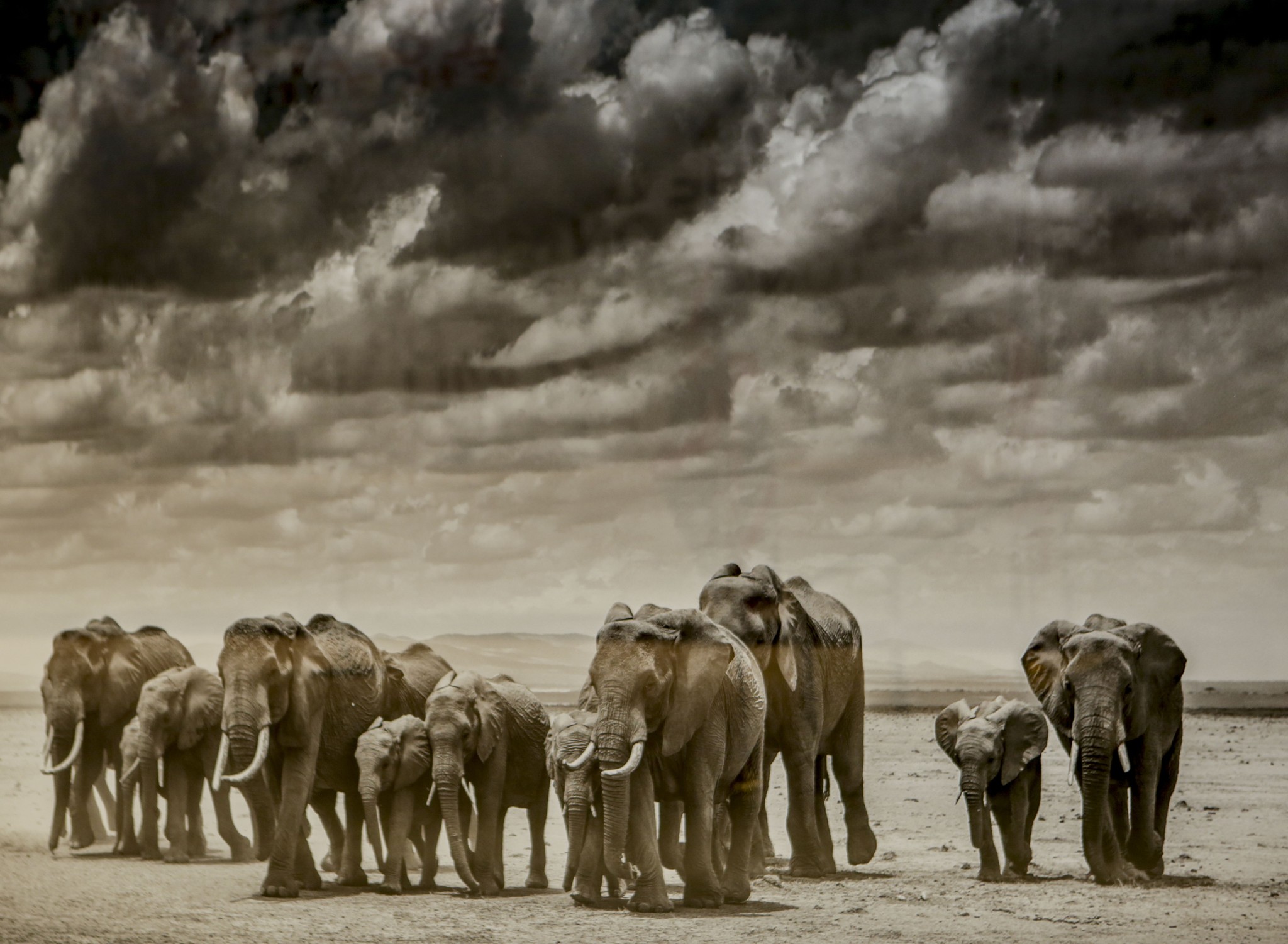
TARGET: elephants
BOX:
[935,695,1049,881]
[1020,614,1188,885]
[41,560,877,914]
